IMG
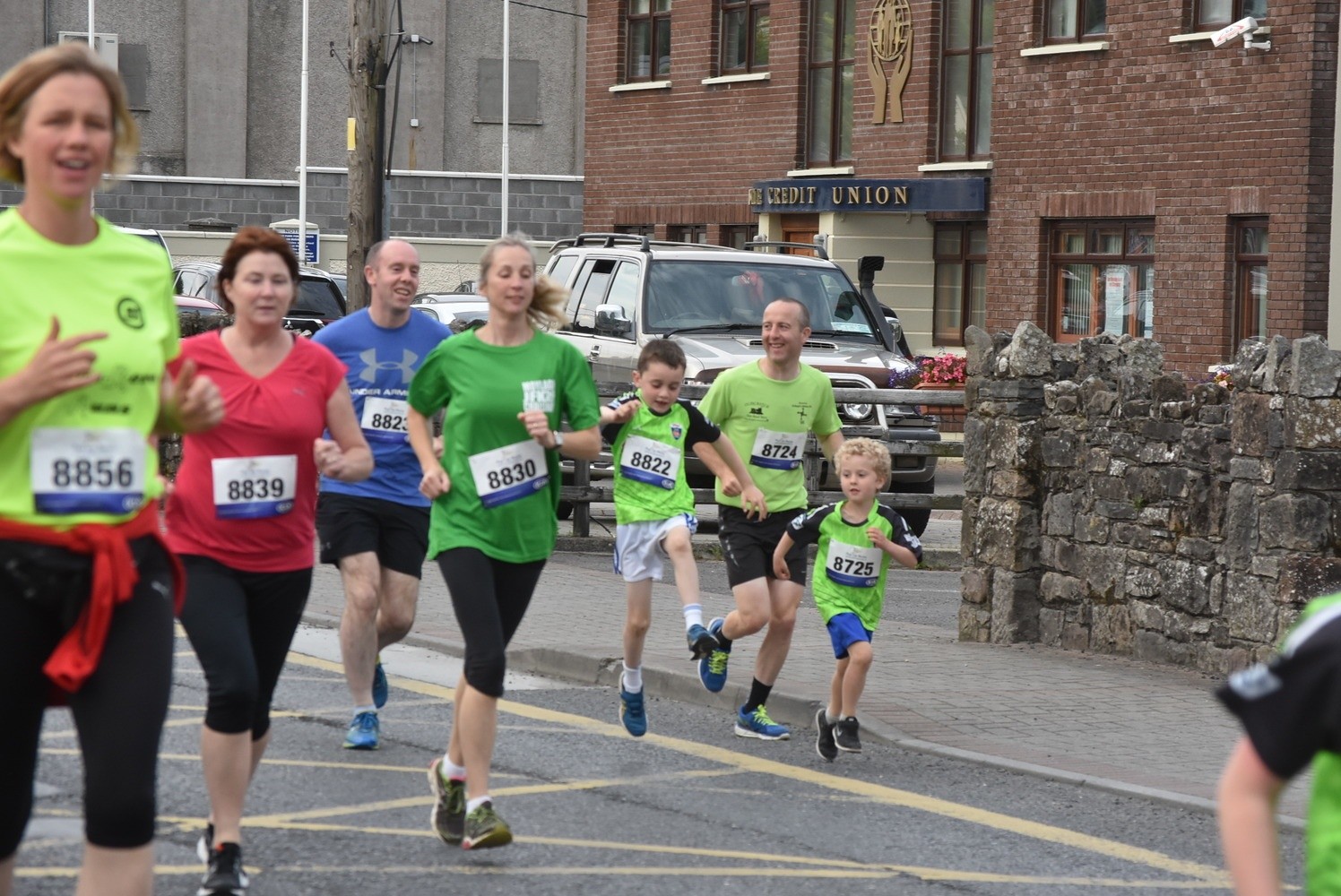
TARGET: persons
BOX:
[310,238,455,751]
[166,224,374,895]
[771,436,925,763]
[0,35,225,895]
[694,298,848,742]
[403,230,602,851]
[598,340,766,737]
[1209,595,1341,895]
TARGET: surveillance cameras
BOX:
[1210,17,1258,49]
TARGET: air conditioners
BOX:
[57,30,121,74]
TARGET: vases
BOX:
[913,382,967,415]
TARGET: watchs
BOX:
[548,430,563,451]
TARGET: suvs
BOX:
[532,231,940,542]
[115,223,173,265]
[174,262,346,339]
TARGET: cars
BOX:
[405,292,493,328]
[173,293,228,338]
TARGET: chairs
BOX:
[728,279,778,317]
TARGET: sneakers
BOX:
[816,709,838,761]
[197,842,250,896]
[428,759,467,846]
[372,655,388,708]
[734,704,790,740]
[461,801,512,850]
[196,823,216,866]
[697,617,732,693]
[686,624,720,660]
[832,716,862,753]
[343,710,380,749]
[619,672,648,740]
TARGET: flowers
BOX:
[887,354,967,389]
